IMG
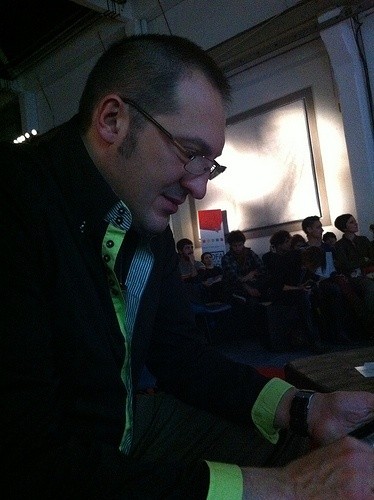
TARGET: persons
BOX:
[176,238,204,283]
[221,230,287,318]
[200,251,227,304]
[262,215,374,354]
[0,33,374,500]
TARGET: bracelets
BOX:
[289,389,316,432]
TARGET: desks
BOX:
[285,348,373,442]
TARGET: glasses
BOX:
[123,99,226,180]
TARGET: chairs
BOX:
[200,285,237,344]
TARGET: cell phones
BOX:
[347,420,374,446]
